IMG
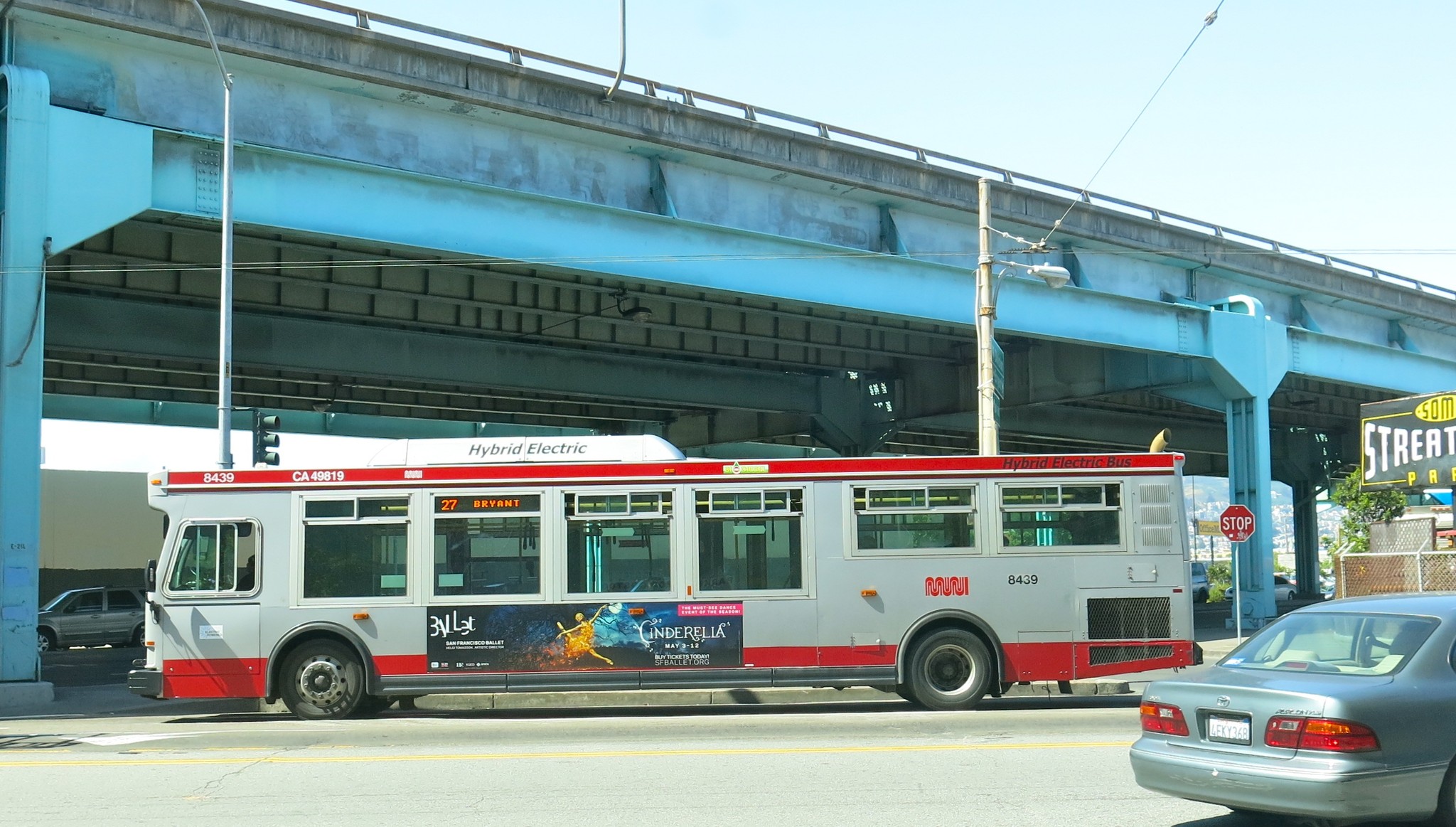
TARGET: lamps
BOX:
[608,288,653,325]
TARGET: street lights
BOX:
[977,253,1070,456]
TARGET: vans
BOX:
[1191,562,1210,603]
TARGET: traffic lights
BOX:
[252,410,280,467]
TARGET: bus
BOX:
[126,427,1204,722]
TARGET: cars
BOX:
[1275,568,1334,600]
[1224,575,1297,601]
[1130,591,1456,827]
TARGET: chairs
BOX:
[1389,631,1416,655]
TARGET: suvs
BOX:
[38,586,146,653]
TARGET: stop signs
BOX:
[1220,504,1256,542]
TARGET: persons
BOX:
[212,556,255,591]
[555,604,613,665]
[1396,620,1425,644]
